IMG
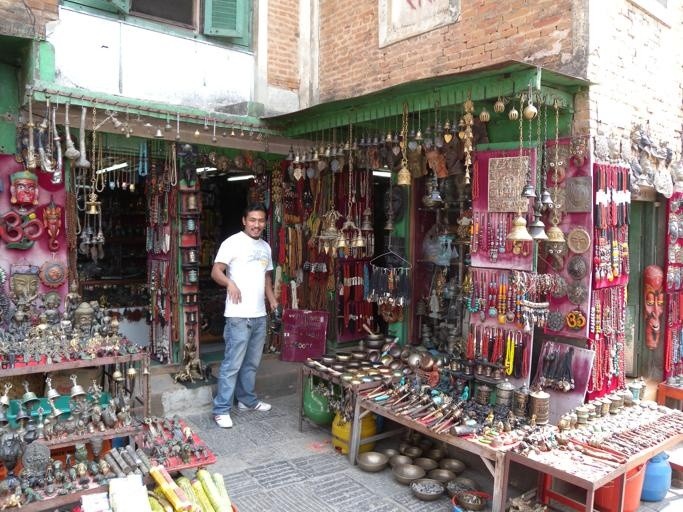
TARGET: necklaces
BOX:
[664,290,682,373]
[467,214,526,379]
[586,222,633,394]
[65,131,179,257]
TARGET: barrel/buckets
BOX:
[640,453,673,502]
[593,462,649,512]
[331,401,378,455]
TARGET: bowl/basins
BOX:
[304,332,446,386]
[356,442,492,512]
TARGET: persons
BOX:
[0,273,115,337]
[209,201,284,429]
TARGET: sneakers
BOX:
[215,415,233,428]
[238,400,271,412]
